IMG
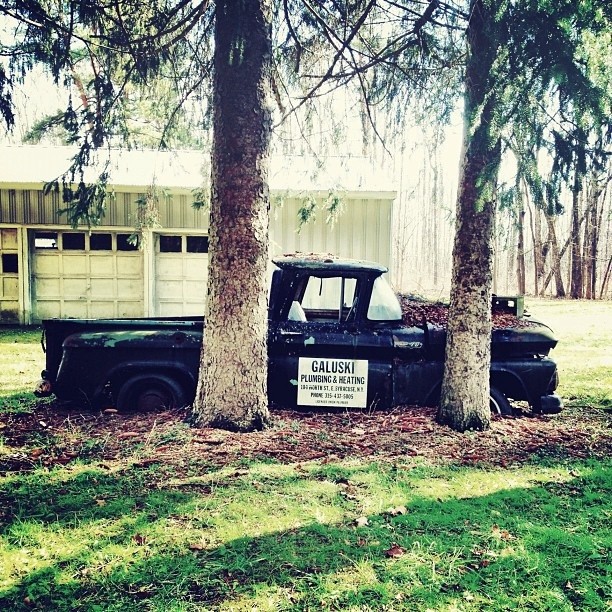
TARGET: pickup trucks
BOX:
[35,252,562,415]
